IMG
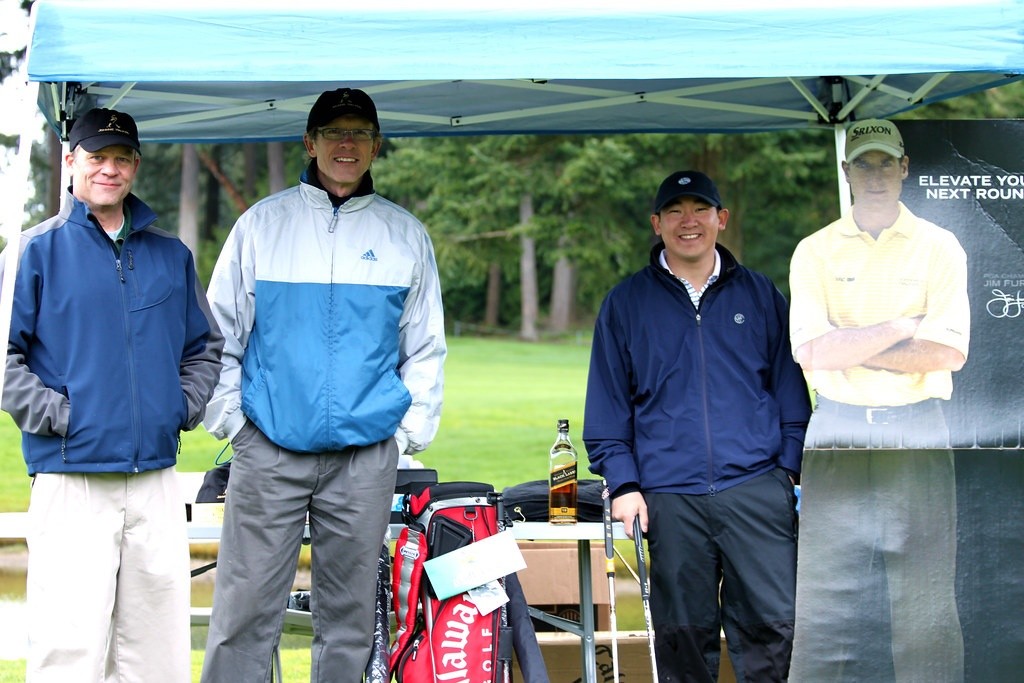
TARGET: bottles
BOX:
[548,418,580,525]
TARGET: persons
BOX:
[203,86,448,682]
[788,118,970,683]
[1,108,227,683]
[581,171,812,683]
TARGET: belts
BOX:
[816,393,943,425]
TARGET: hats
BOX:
[69,106,142,157]
[306,88,381,133]
[844,117,906,165]
[654,170,723,218]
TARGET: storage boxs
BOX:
[517,541,612,635]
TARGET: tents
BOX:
[20,3,1024,208]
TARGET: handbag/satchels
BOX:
[501,479,605,523]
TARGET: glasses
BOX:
[314,126,376,142]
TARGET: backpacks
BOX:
[393,479,549,683]
